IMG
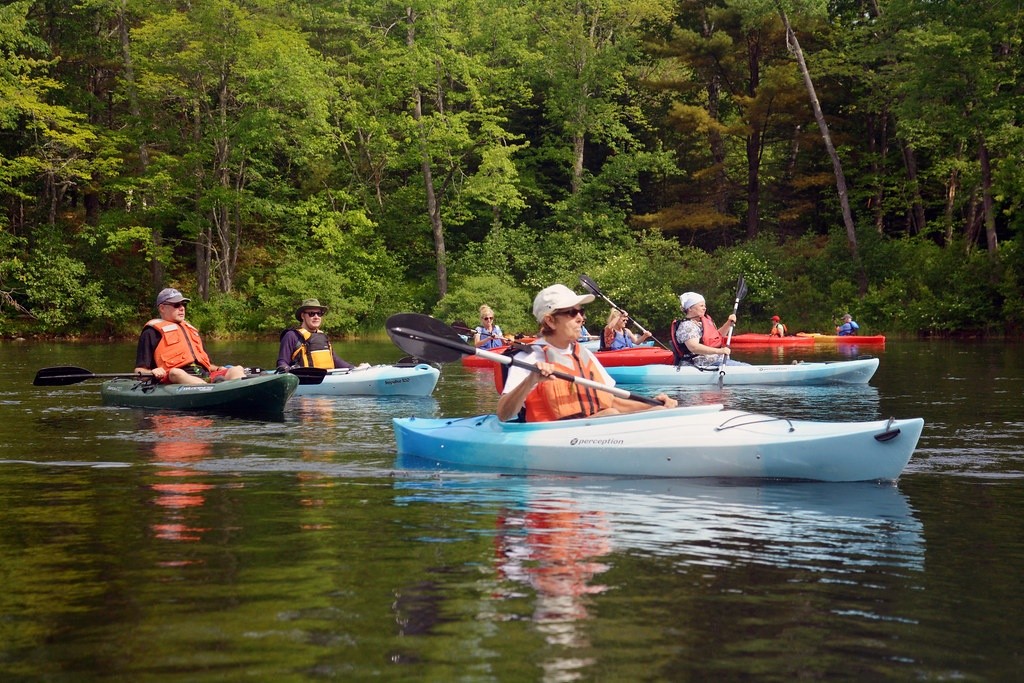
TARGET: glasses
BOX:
[551,307,585,317]
[619,319,628,322]
[302,312,323,317]
[163,303,187,308]
[481,317,494,321]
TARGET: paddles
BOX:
[580,272,670,350]
[31,363,332,388]
[325,356,434,377]
[720,272,748,375]
[384,313,665,405]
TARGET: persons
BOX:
[277,300,371,372]
[577,316,592,342]
[493,284,678,422]
[671,292,748,365]
[835,314,859,336]
[600,308,652,350]
[771,316,787,338]
[135,288,245,384]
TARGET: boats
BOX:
[224,364,441,398]
[602,357,880,390]
[579,338,657,355]
[463,345,514,369]
[797,332,887,349]
[100,368,301,418]
[722,332,816,351]
[590,345,674,369]
[392,404,928,488]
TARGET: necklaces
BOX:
[473,304,513,350]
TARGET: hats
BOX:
[680,292,705,311]
[295,298,327,322]
[532,284,595,324]
[841,314,853,319]
[771,316,780,324]
[157,288,191,306]
